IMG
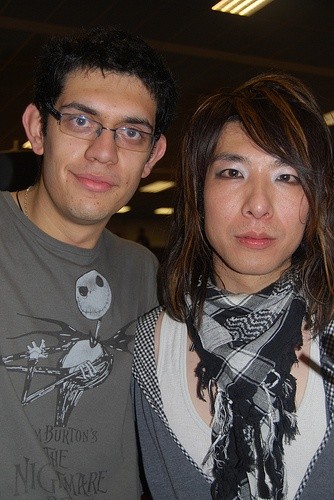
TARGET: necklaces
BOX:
[17,184,32,218]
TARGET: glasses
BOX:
[41,100,161,155]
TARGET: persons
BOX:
[0,26,177,500]
[129,71,334,500]
